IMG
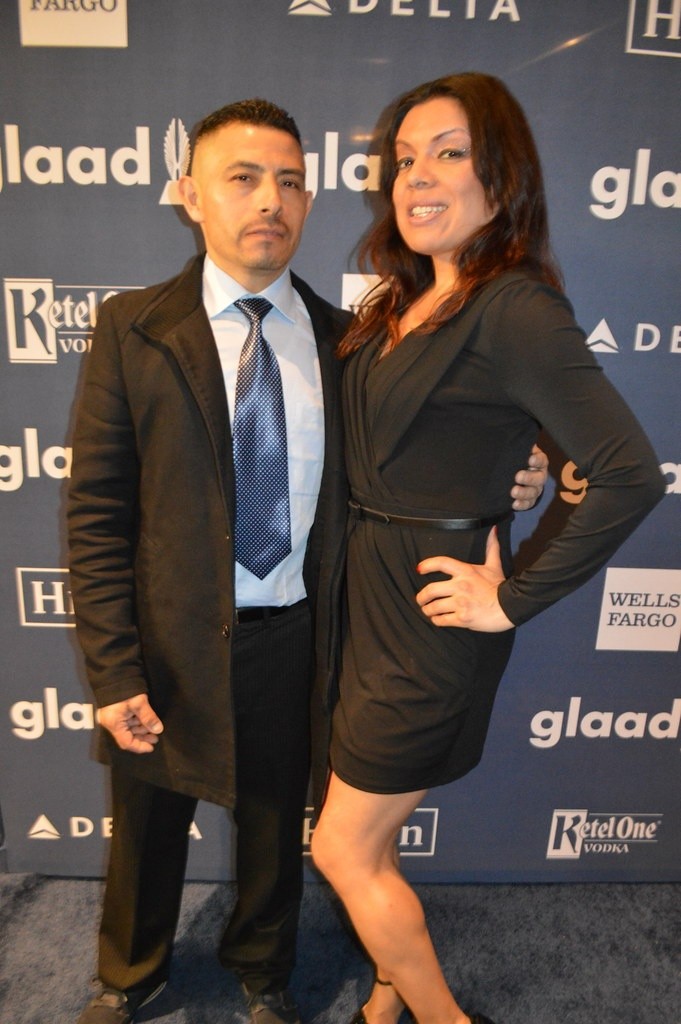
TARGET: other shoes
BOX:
[348,977,413,1024]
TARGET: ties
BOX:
[231,297,291,582]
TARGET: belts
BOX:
[234,606,286,625]
[348,500,513,531]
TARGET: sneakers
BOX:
[242,974,300,1024]
[77,978,167,1024]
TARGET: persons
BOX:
[60,94,553,1024]
[307,69,670,1024]
